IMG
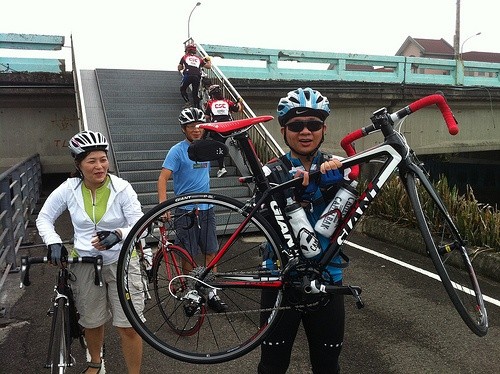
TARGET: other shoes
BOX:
[182,102,195,109]
[206,294,231,312]
[216,167,227,177]
[183,298,203,316]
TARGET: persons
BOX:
[204,85,241,178]
[157,107,229,312]
[178,44,211,109]
[35,131,148,374]
[255,87,351,374]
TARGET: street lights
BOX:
[188,2,201,43]
[461,32,481,59]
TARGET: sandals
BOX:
[79,358,106,374]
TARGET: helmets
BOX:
[276,86,332,126]
[185,44,196,54]
[67,130,111,155]
[208,84,222,95]
[177,107,208,125]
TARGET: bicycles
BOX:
[115,92,488,365]
[142,208,205,337]
[199,67,211,111]
[227,99,248,149]
[19,254,103,374]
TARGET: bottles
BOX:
[285,197,321,258]
[142,244,154,271]
[314,180,359,238]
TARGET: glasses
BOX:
[281,120,324,132]
[186,122,202,129]
